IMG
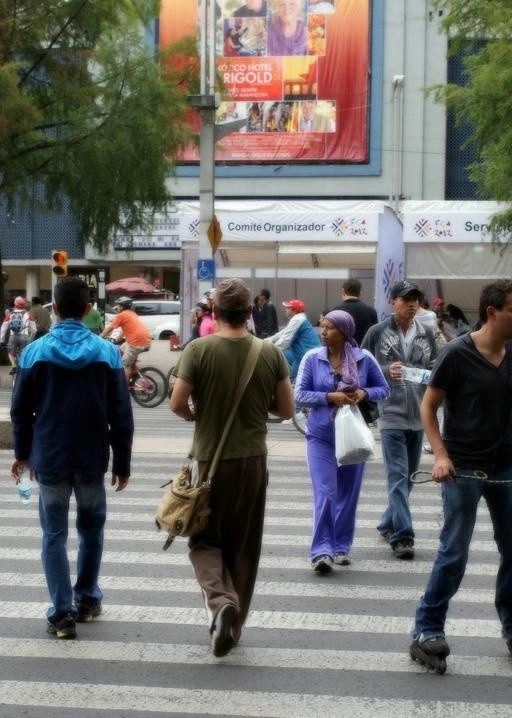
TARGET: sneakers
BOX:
[393,539,415,559]
[382,531,393,542]
[311,554,333,572]
[46,606,77,638]
[9,365,17,375]
[210,603,238,657]
[75,598,103,623]
[335,552,351,565]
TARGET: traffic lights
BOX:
[53,250,67,277]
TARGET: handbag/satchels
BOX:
[155,455,214,550]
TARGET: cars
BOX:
[42,303,54,319]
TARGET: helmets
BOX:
[115,297,133,307]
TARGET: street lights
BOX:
[187,90,221,302]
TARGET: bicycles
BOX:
[123,358,157,403]
[101,335,168,409]
[292,397,313,435]
[1,343,21,390]
[264,403,287,426]
[166,345,183,388]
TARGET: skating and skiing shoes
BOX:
[409,631,450,675]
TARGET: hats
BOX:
[211,278,250,311]
[389,281,424,301]
[282,300,305,312]
[433,298,443,308]
[14,296,25,308]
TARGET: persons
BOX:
[252,279,472,424]
[224,0,334,56]
[174,288,217,352]
[361,280,441,560]
[0,297,51,375]
[409,279,511,672]
[174,277,296,657]
[9,276,134,638]
[215,100,335,133]
[83,289,152,383]
[294,310,390,573]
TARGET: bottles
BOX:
[393,367,435,387]
[20,467,33,506]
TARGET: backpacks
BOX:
[9,310,27,333]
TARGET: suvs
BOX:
[104,300,181,341]
